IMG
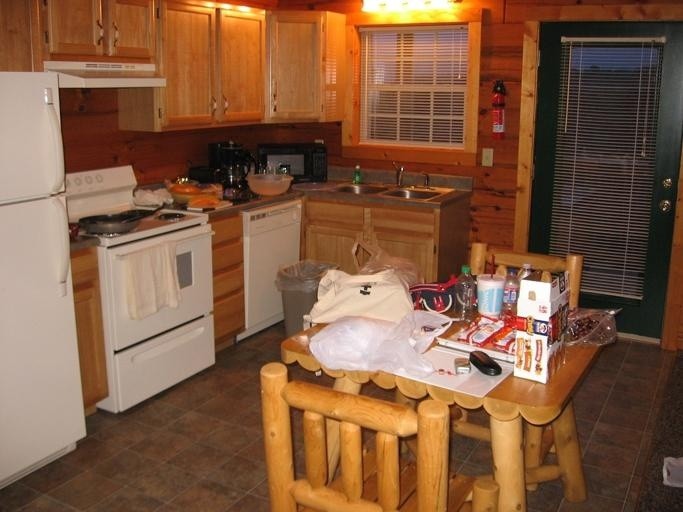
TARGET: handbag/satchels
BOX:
[306,268,414,324]
[409,276,466,318]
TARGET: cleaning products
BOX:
[352,165,364,184]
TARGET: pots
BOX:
[77,207,164,233]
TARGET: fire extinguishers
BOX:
[490,79,506,140]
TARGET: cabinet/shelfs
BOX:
[37,1,160,63]
[0,0,43,72]
[264,10,346,124]
[305,198,471,298]
[118,0,270,131]
[70,248,108,417]
[207,214,245,352]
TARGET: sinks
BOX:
[381,188,441,200]
[333,184,389,195]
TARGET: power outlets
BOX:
[482,149,493,167]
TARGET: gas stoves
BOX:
[77,210,208,248]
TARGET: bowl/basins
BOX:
[169,177,222,205]
[245,174,293,196]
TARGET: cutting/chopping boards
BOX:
[392,345,514,399]
[187,202,233,212]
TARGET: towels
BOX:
[124,241,180,318]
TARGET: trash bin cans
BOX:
[277,259,339,338]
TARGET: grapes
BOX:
[569,318,612,344]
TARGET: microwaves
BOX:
[257,143,327,185]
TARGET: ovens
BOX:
[92,225,216,415]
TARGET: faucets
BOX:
[422,172,431,186]
[391,160,405,186]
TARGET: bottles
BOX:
[352,164,361,184]
[503,264,533,314]
[453,266,481,322]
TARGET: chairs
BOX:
[452,242,587,492]
[261,361,499,512]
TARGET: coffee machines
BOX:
[208,142,261,204]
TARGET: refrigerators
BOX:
[1,73,87,491]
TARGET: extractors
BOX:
[42,60,167,90]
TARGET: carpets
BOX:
[638,347,683,512]
